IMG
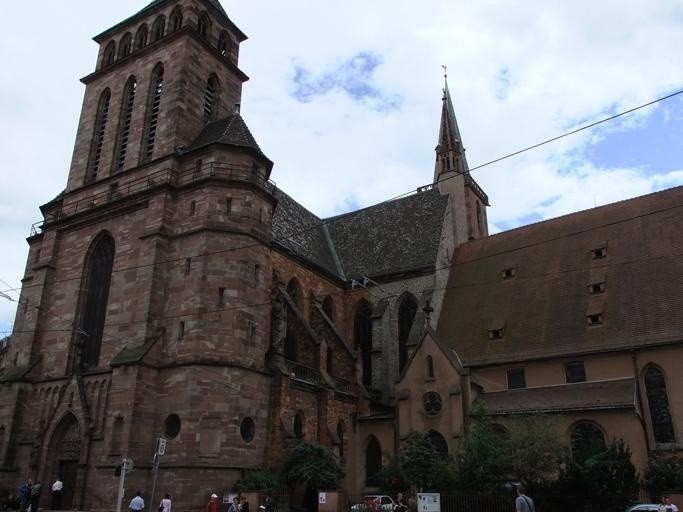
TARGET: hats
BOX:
[348,493,402,512]
[624,503,680,512]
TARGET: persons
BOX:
[19,477,63,512]
[392,492,408,512]
[657,496,680,512]
[515,485,535,512]
[159,493,172,512]
[205,494,218,512]
[228,494,275,512]
[128,490,144,512]
